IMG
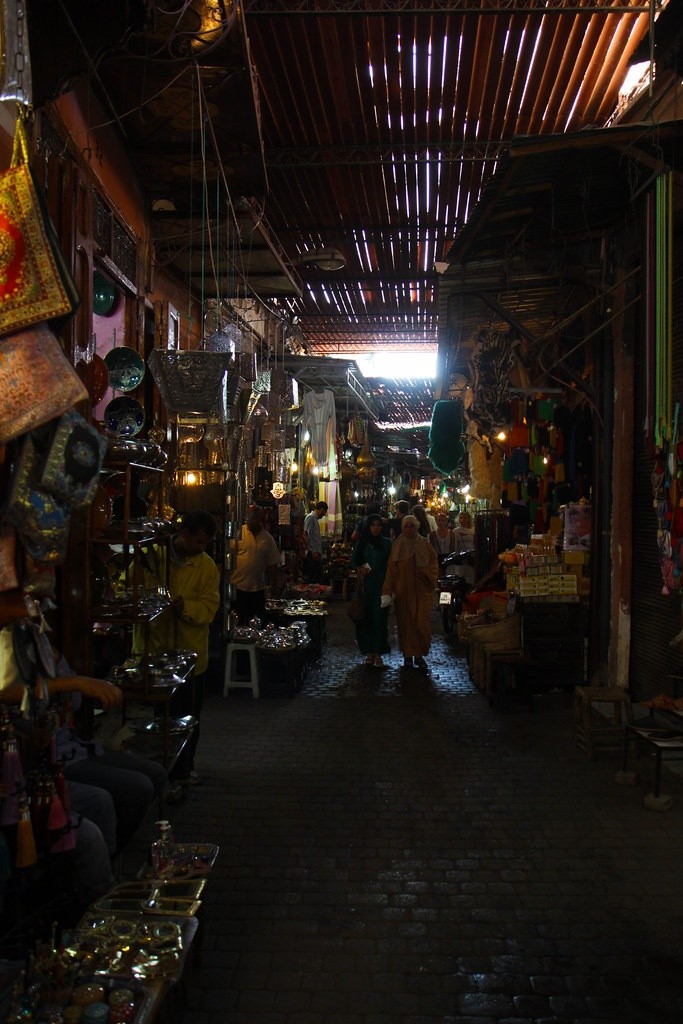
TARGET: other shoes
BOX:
[625,716,671,732]
[672,697,683,712]
[403,657,413,668]
[371,656,386,668]
[639,693,673,711]
[644,728,683,741]
[414,658,429,669]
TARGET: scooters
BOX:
[437,547,479,635]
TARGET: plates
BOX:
[92,270,115,316]
[93,583,171,619]
[110,516,172,540]
[104,346,145,392]
[106,648,200,691]
[129,713,199,737]
[91,353,107,407]
[104,395,145,439]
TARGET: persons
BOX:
[229,511,280,627]
[351,515,393,666]
[0,626,169,897]
[352,501,475,610]
[382,516,438,669]
[302,501,327,583]
[116,510,220,785]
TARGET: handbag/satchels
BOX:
[346,571,371,622]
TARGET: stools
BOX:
[486,651,535,714]
[622,716,683,798]
[575,686,634,763]
[329,578,348,602]
[223,643,260,699]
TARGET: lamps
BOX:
[284,245,346,273]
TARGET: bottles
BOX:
[107,435,171,469]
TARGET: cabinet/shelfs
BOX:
[80,459,197,820]
[517,601,590,695]
[168,464,236,652]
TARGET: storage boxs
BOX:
[502,533,585,597]
